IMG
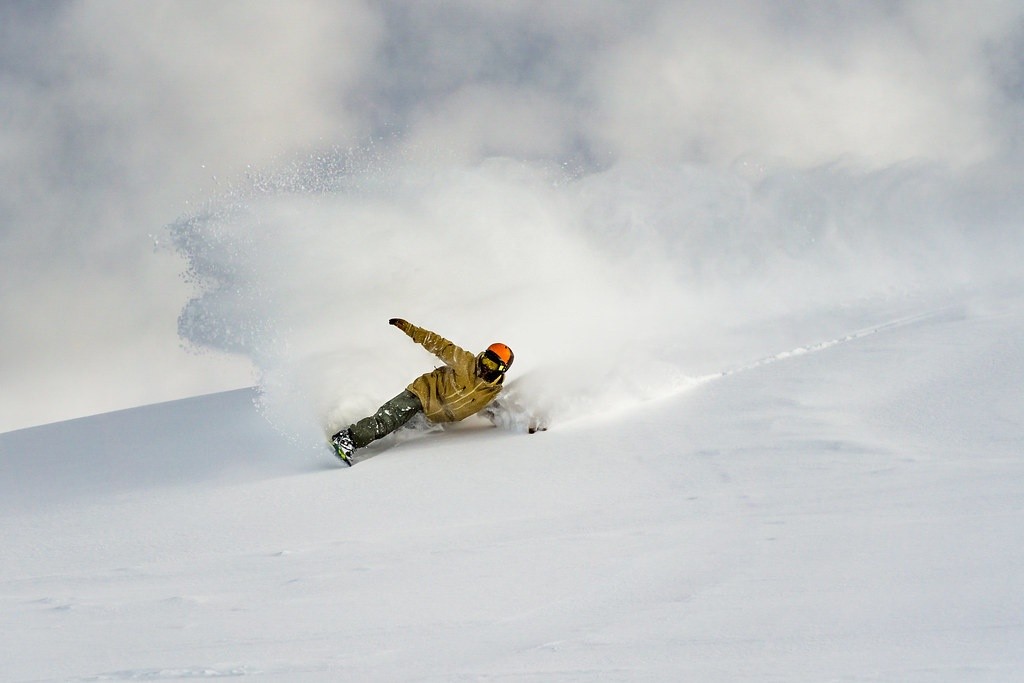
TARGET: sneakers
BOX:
[332,429,358,465]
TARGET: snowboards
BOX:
[329,437,353,469]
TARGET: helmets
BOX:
[479,343,514,382]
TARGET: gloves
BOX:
[389,318,405,329]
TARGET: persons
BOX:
[331,319,548,467]
